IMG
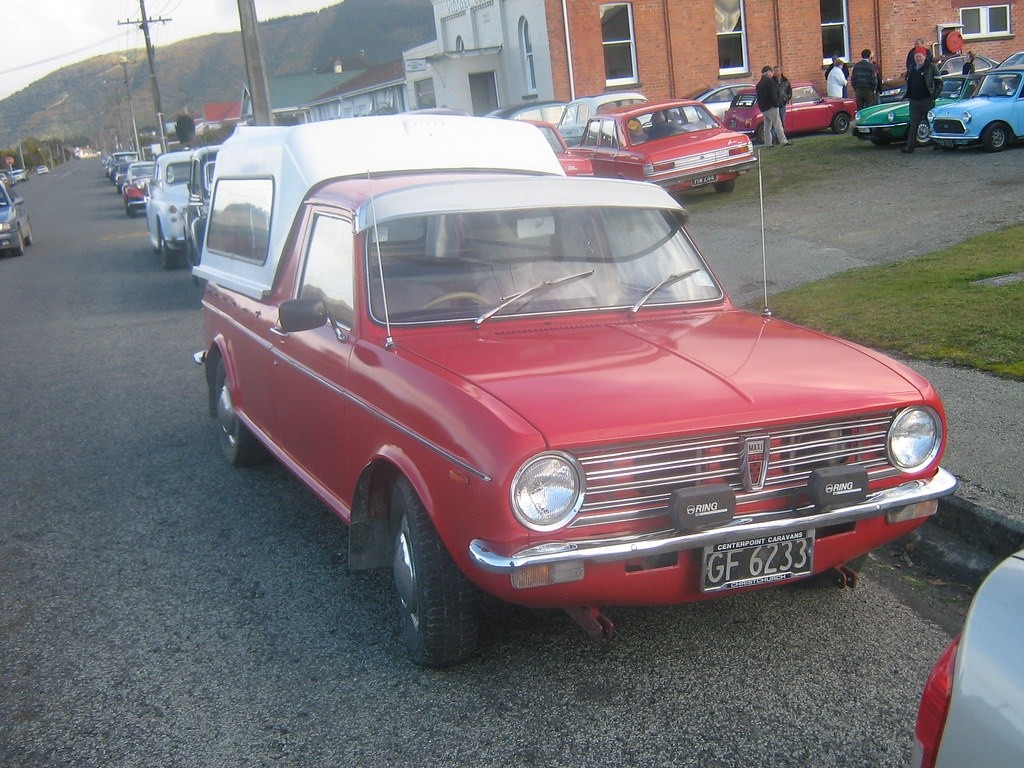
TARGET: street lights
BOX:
[156,113,167,153]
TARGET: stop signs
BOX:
[5,157,14,166]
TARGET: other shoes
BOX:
[766,146,774,148]
[901,148,913,153]
[934,145,940,150]
[784,143,791,147]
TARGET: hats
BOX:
[914,48,926,55]
[774,65,781,70]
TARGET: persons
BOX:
[771,65,793,145]
[629,108,687,144]
[906,39,933,70]
[869,50,882,105]
[827,59,848,98]
[962,50,976,75]
[851,49,877,112]
[756,65,791,149]
[825,55,849,98]
[900,46,944,153]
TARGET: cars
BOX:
[915,545,1024,768]
[118,161,155,218]
[880,53,1001,104]
[671,82,757,124]
[0,169,28,187]
[928,51,1024,152]
[0,180,34,257]
[569,98,758,194]
[483,101,571,135]
[721,81,857,144]
[516,119,595,178]
[133,149,192,269]
[852,71,1009,146]
[192,113,958,670]
[181,145,222,290]
[102,152,140,191]
[37,165,49,174]
[545,92,654,146]
